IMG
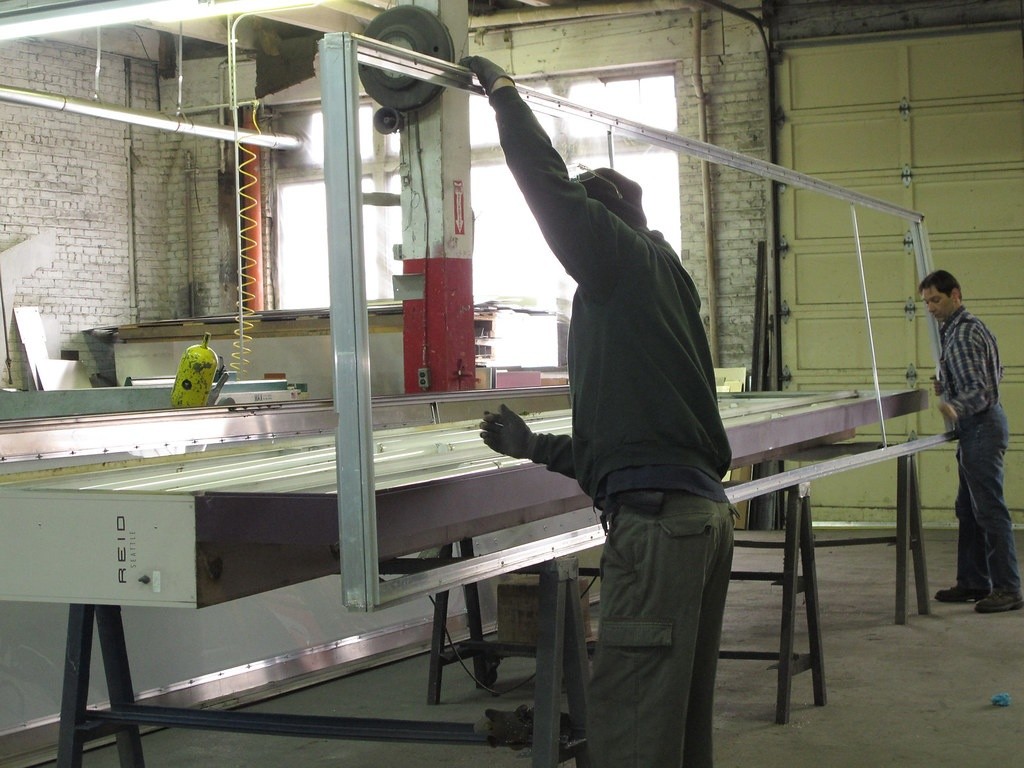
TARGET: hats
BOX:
[575,167,647,227]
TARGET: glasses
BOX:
[566,163,624,200]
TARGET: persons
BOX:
[457,55,740,768]
[920,270,1024,614]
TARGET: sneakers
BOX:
[935,583,990,602]
[974,589,1024,613]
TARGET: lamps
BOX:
[0,1,318,149]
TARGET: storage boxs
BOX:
[497,575,592,646]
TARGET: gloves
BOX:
[458,55,516,95]
[480,403,539,459]
